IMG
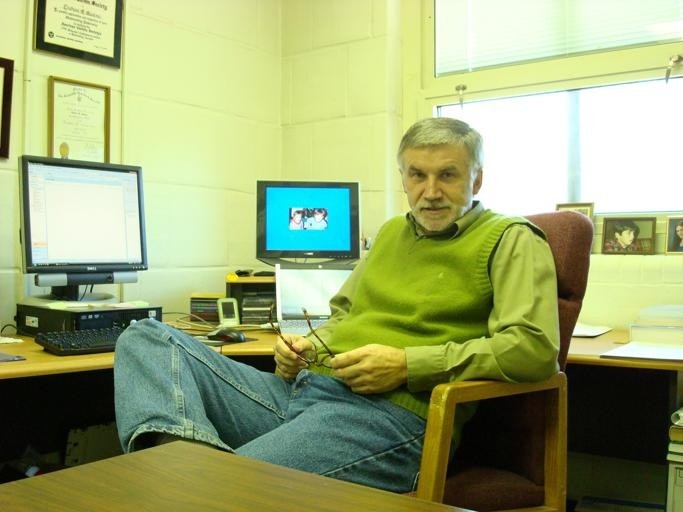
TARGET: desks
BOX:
[0,322,683,411]
[0,440,480,512]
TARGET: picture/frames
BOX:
[555,202,594,255]
[600,216,656,255]
[0,57,14,158]
[664,215,683,256]
[32,0,123,69]
[46,75,110,165]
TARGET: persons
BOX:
[306,209,327,230]
[289,208,306,228]
[670,221,682,252]
[604,221,642,250]
[110,116,561,492]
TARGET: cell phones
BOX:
[217,297,240,328]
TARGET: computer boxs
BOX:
[15,297,163,340]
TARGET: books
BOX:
[667,404,682,464]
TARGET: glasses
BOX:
[269,302,336,370]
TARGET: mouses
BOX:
[209,324,246,343]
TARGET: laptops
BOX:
[275,262,357,340]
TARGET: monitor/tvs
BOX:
[17,154,149,301]
[256,179,361,265]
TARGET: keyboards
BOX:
[34,326,126,356]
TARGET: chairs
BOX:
[398,211,595,512]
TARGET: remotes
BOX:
[236,268,254,276]
